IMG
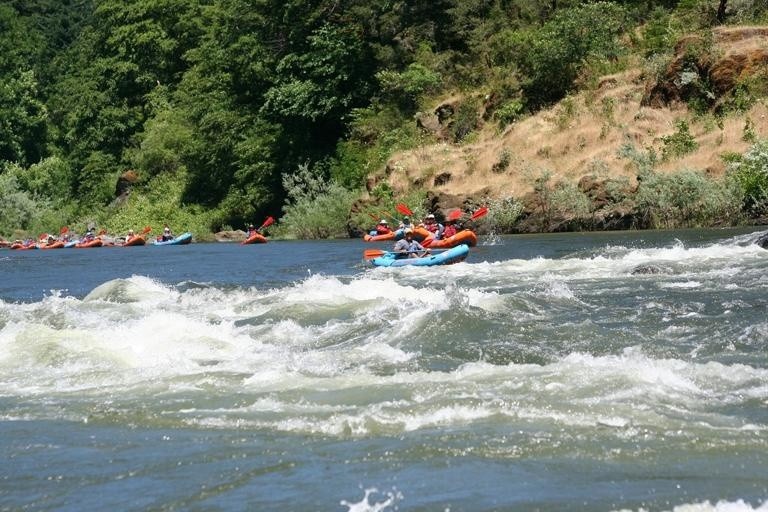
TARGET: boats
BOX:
[238,232,268,245]
[360,222,478,267]
[0,233,194,252]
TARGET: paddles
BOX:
[58,226,68,239]
[460,208,487,227]
[396,204,421,223]
[380,210,402,225]
[139,228,150,236]
[255,217,273,232]
[364,249,447,258]
[368,214,391,233]
[446,210,459,221]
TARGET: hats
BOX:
[247,225,254,229]
[427,214,435,219]
[403,228,413,236]
[380,219,389,224]
[403,216,409,220]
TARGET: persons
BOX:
[22,226,173,247]
[370,214,468,258]
[246,225,257,239]
